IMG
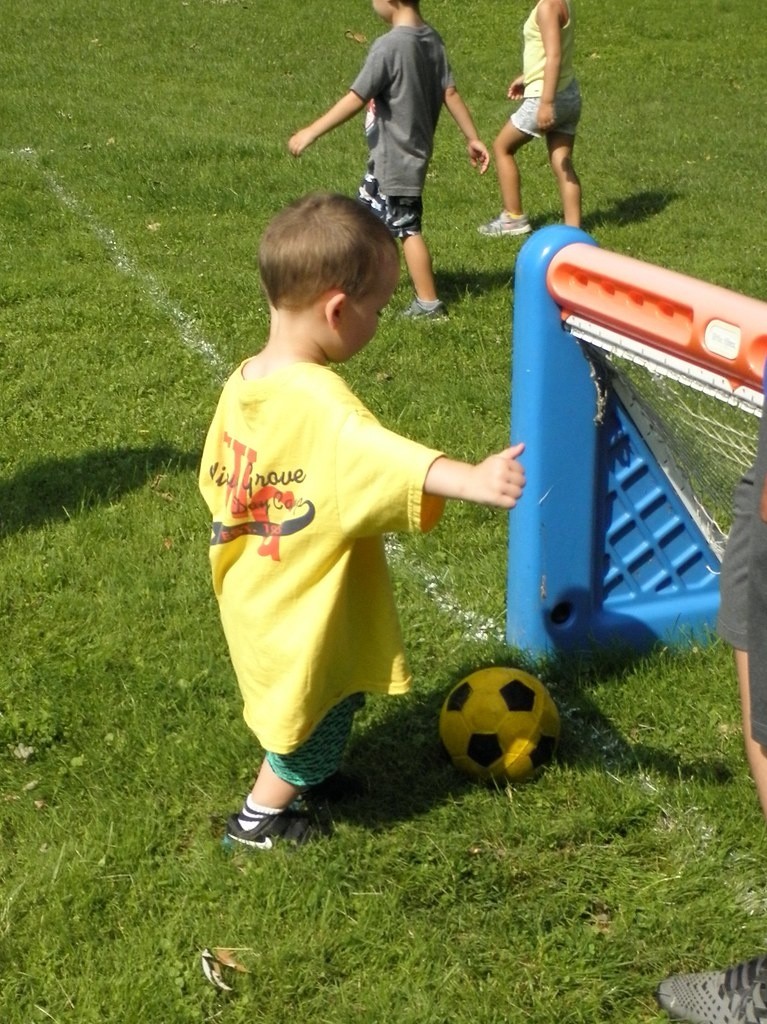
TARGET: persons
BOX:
[286,0,492,323]
[653,356,766,1024]
[194,189,526,854]
[476,1,585,235]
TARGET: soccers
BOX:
[438,664,559,787]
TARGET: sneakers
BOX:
[476,211,532,237]
[403,300,450,325]
[224,807,332,850]
[658,954,767,1024]
